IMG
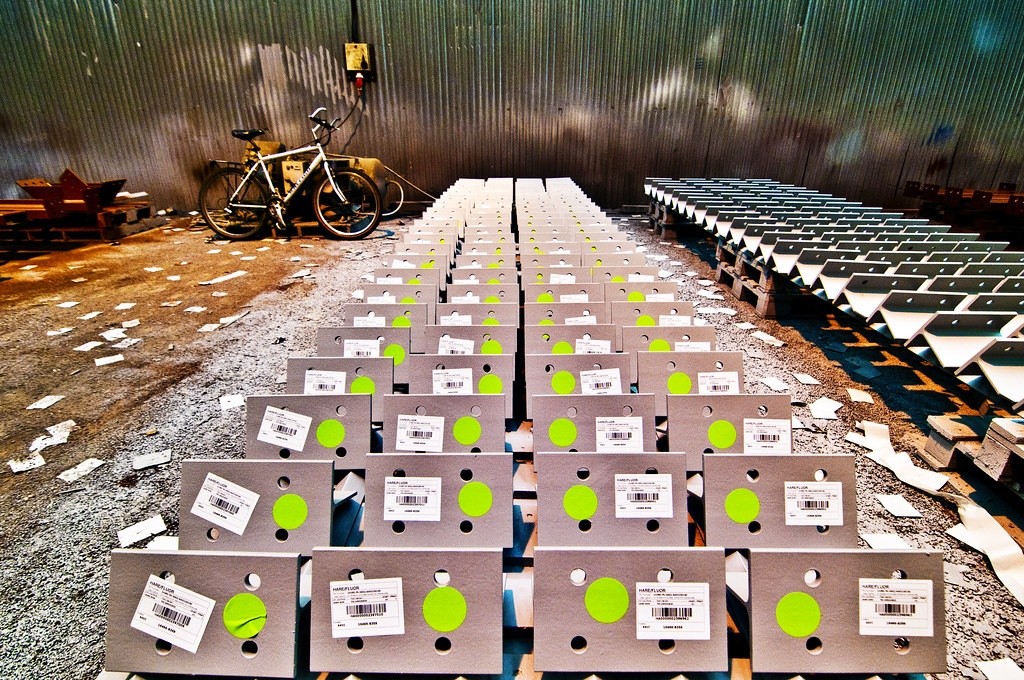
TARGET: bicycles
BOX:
[198,106,386,240]
